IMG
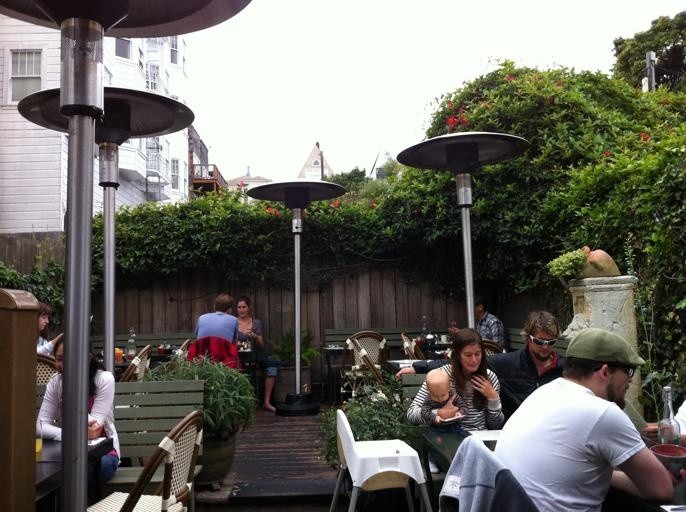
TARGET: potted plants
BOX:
[544,246,621,280]
[267,328,323,404]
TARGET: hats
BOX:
[566,328,646,366]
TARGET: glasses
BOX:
[528,334,556,346]
[625,365,635,377]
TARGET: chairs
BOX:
[329,409,433,512]
[439,436,541,512]
[36,339,205,512]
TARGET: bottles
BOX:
[237,337,252,352]
[157,341,179,353]
[656,385,681,446]
[125,327,137,362]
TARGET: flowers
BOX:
[130,349,257,489]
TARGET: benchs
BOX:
[90,332,195,367]
[323,329,570,412]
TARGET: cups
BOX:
[441,334,450,342]
[34,419,44,454]
[648,442,686,478]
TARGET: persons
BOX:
[407,328,504,431]
[396,310,567,427]
[37,304,64,357]
[448,302,508,349]
[421,369,468,435]
[38,335,122,506]
[237,296,278,413]
[493,327,674,511]
[194,293,239,345]
[640,400,686,507]
